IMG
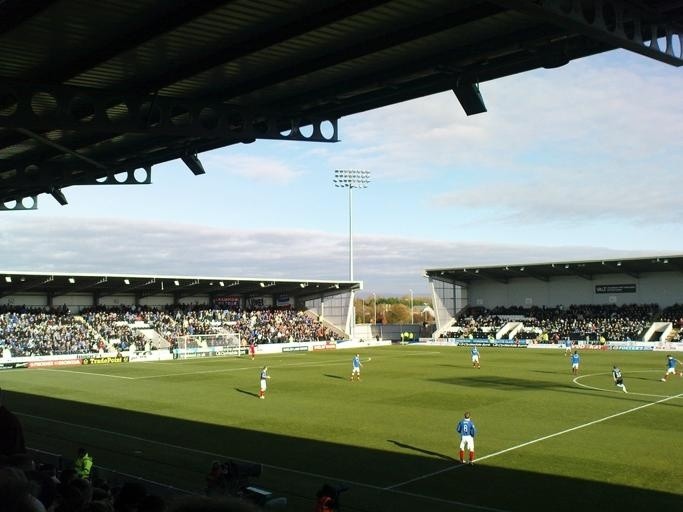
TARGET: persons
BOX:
[569,350,580,376]
[660,354,683,383]
[441,303,682,357]
[376,333,380,341]
[257,366,272,400]
[195,299,344,344]
[457,410,477,467]
[470,345,481,369]
[399,329,414,342]
[1,303,104,357]
[173,341,178,359]
[249,340,255,360]
[349,352,363,382]
[0,405,350,512]
[81,302,200,352]
[612,364,628,394]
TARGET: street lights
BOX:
[371,292,377,326]
[332,168,372,281]
[409,288,414,325]
